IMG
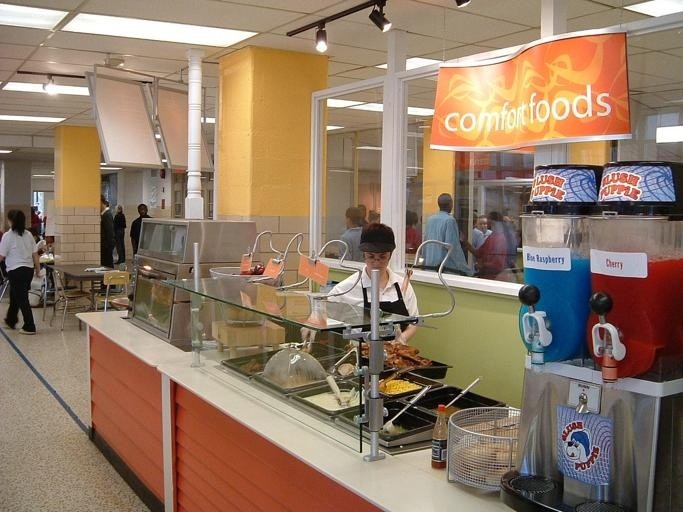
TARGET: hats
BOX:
[359,223,394,253]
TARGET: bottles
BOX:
[430,404,449,470]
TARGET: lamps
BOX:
[454,0,470,7]
[315,21,328,52]
[368,1,392,33]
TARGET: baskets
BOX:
[447,407,521,492]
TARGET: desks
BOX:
[74,309,522,512]
[48,263,115,309]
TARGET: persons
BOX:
[101,193,156,303]
[339,204,380,263]
[406,209,422,269]
[421,192,472,277]
[458,208,519,279]
[299,222,420,358]
[0,206,55,335]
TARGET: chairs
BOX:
[94,270,130,311]
[42,265,76,320]
[51,270,92,332]
